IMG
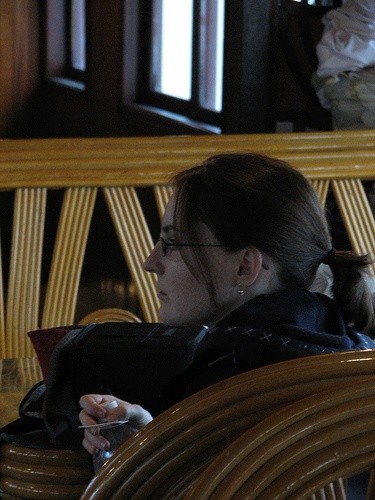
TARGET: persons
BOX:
[74,147,374,500]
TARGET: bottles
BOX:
[93,399,139,476]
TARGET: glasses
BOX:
[159,225,271,271]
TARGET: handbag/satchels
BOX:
[45,323,210,435]
